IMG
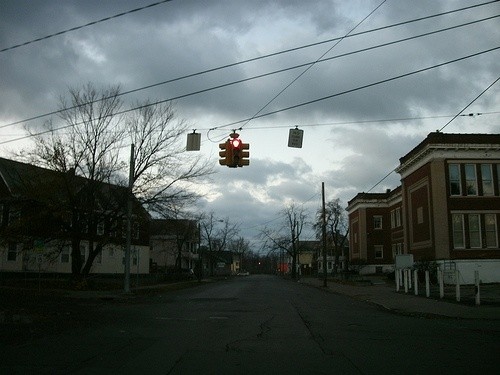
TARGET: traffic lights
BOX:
[220,134,250,167]
